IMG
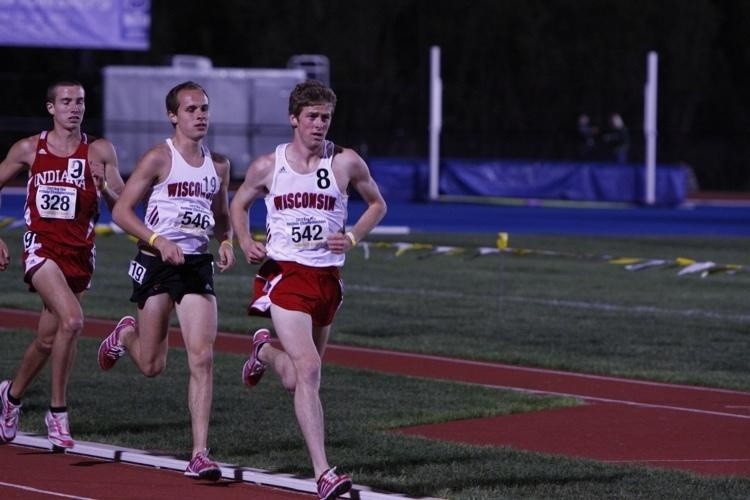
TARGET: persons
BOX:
[0,80,125,448]
[98,81,235,480]
[229,80,388,500]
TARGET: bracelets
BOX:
[99,181,107,191]
[221,240,233,248]
[345,232,356,247]
[149,233,160,247]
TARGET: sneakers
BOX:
[96,315,137,372]
[0,379,23,442]
[44,408,75,449]
[316,466,352,500]
[242,327,273,388]
[183,448,222,482]
